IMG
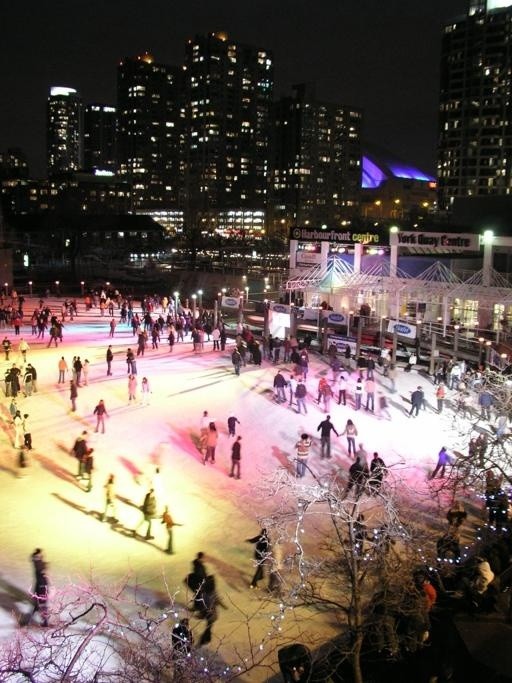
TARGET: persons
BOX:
[230,288,427,420]
[432,313,508,481]
[169,549,229,682]
[247,529,285,599]
[339,479,511,682]
[19,549,54,629]
[276,643,315,683]
[56,345,184,556]
[199,410,243,481]
[84,288,230,359]
[0,286,78,348]
[289,415,387,502]
[0,336,38,470]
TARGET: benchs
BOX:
[396,361,429,372]
